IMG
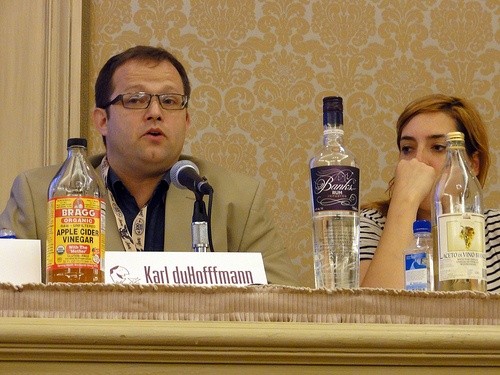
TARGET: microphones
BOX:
[170,160,214,195]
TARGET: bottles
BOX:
[46,139,106,283]
[310,96,360,289]
[404,220,434,291]
[430,131,487,292]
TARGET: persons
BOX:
[2,44,296,287]
[358,92,500,295]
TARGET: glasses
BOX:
[104,92,187,110]
